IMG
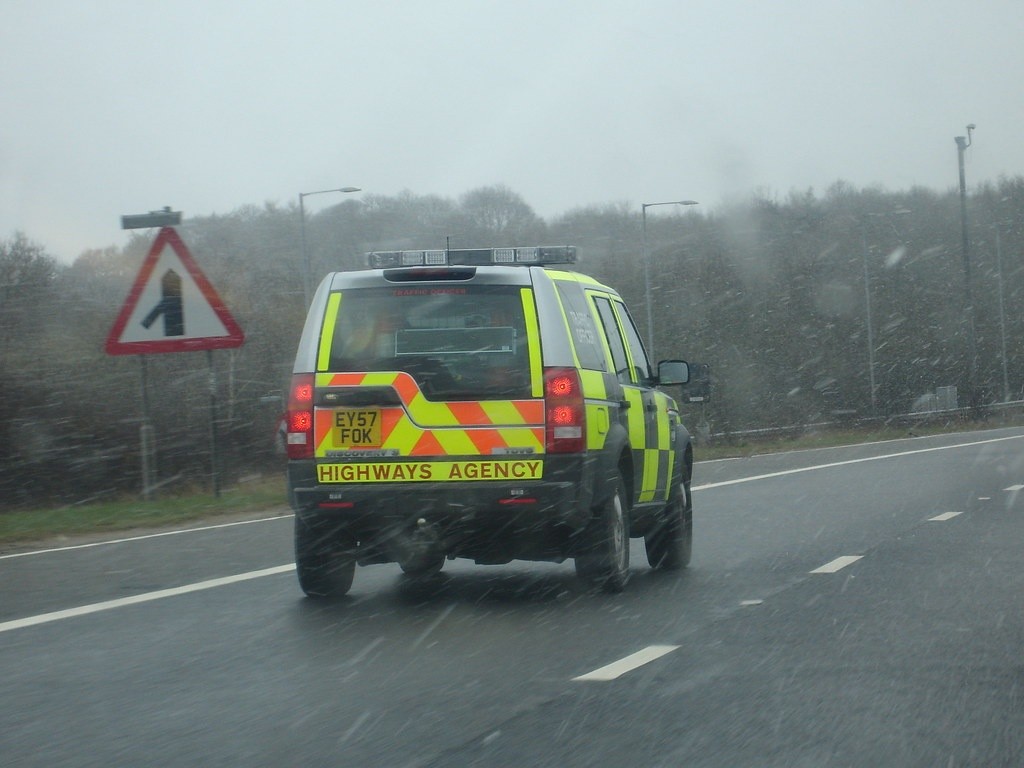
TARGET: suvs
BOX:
[272,244,699,606]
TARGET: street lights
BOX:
[639,199,701,376]
[954,121,978,286]
[297,186,362,314]
